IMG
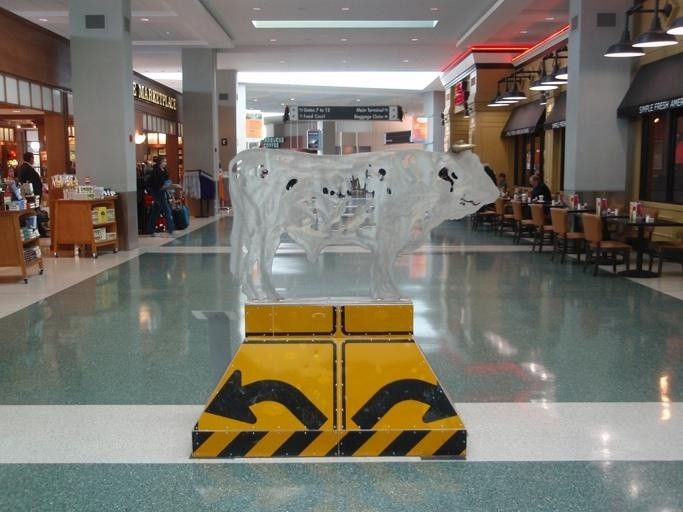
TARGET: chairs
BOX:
[472,194,682,278]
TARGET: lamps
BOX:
[603,1,683,59]
[486,48,568,106]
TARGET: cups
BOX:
[514,193,544,203]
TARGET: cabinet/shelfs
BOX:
[0,209,44,284]
[52,198,120,258]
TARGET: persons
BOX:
[484,164,497,186]
[15,151,50,239]
[144,154,179,238]
[496,173,507,190]
[528,175,552,218]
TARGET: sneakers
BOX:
[150,230,183,238]
